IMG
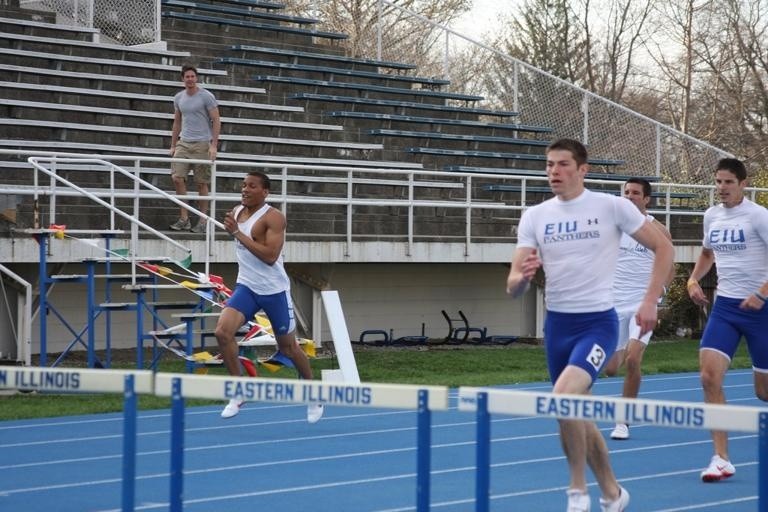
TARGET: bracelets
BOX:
[752,290,767,304]
[170,143,176,147]
[661,284,668,297]
[231,230,239,235]
[684,278,697,288]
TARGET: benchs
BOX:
[0,0,706,239]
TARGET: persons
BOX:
[213,170,326,425]
[501,136,677,511]
[683,156,768,486]
[167,62,221,236]
[601,175,675,442]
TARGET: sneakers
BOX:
[597,485,630,512]
[609,422,632,441]
[306,404,324,424]
[700,455,737,482]
[169,216,191,230]
[190,222,206,233]
[565,489,591,512]
[221,397,244,419]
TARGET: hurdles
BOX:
[458,385,768,512]
[0,366,154,512]
[156,370,448,512]
